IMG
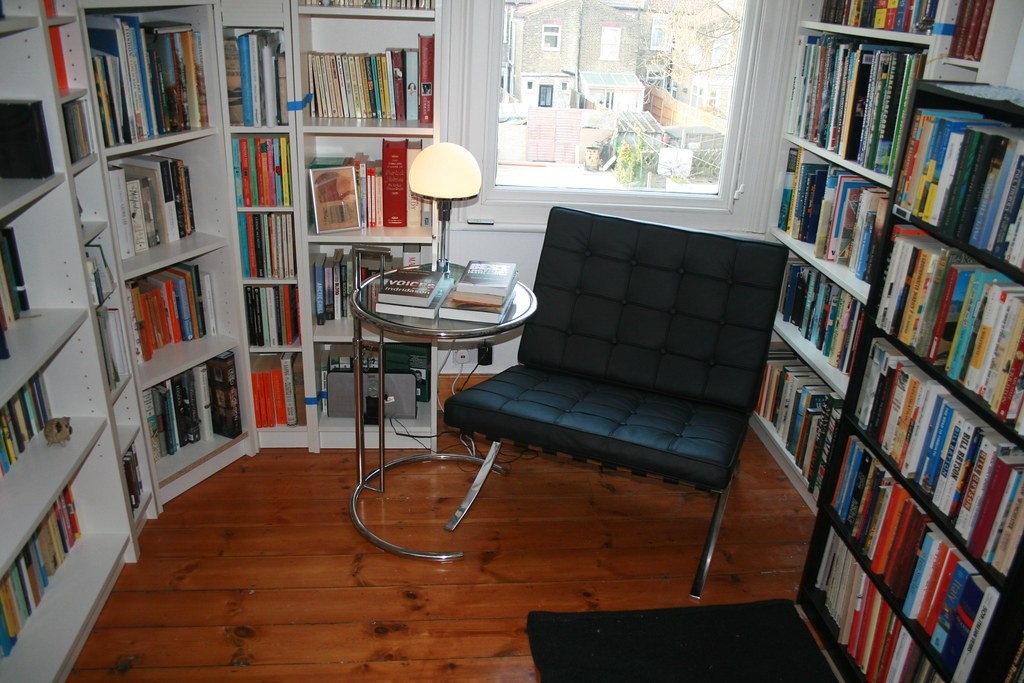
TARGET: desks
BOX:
[347,262,539,560]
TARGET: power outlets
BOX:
[453,347,478,364]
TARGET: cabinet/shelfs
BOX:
[748,0,1023,519]
[793,78,1024,683]
[0,0,442,683]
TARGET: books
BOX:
[122,351,242,519]
[232,133,437,429]
[0,97,82,683]
[108,153,196,258]
[82,0,439,148]
[753,0,1024,683]
[375,259,518,325]
[84,245,217,392]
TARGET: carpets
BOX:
[526,599,840,683]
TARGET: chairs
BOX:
[442,206,790,600]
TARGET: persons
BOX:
[415,370,426,395]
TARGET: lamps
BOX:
[408,142,483,282]
